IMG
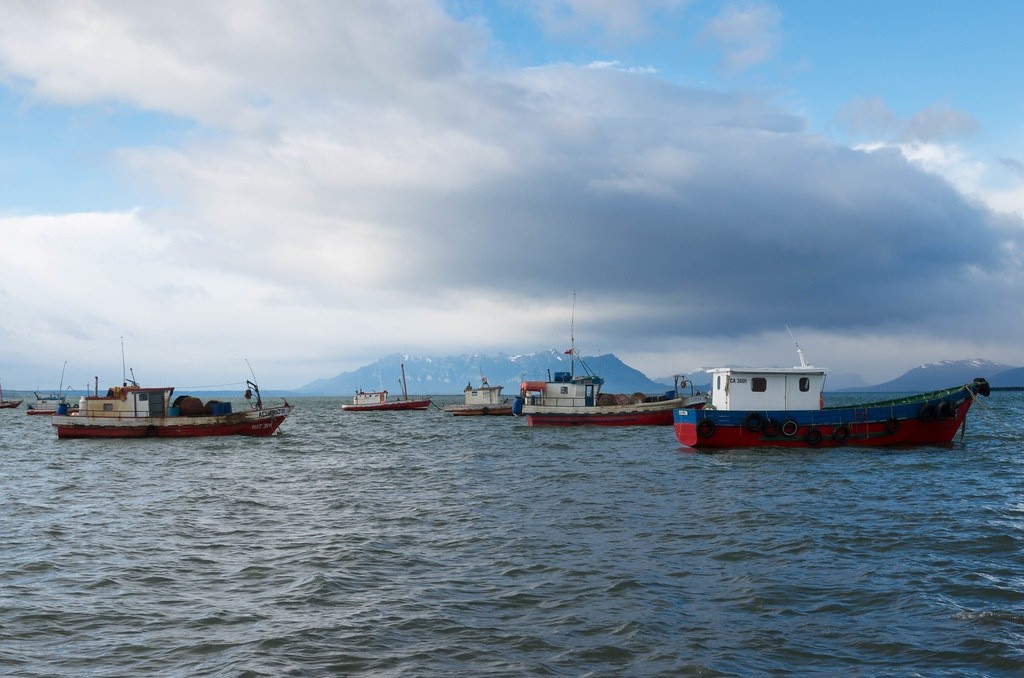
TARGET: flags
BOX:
[565,349,571,354]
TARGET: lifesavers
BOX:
[146,425,157,436]
[696,418,717,437]
[482,407,489,414]
[781,419,798,437]
[745,413,765,433]
[937,401,949,422]
[884,416,900,433]
[919,404,937,423]
[832,425,851,443]
[762,419,781,437]
[805,428,823,446]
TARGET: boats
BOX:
[444,386,512,416]
[27,397,80,415]
[0,395,23,409]
[52,382,293,436]
[673,342,990,449]
[510,339,706,426]
[340,363,431,410]
